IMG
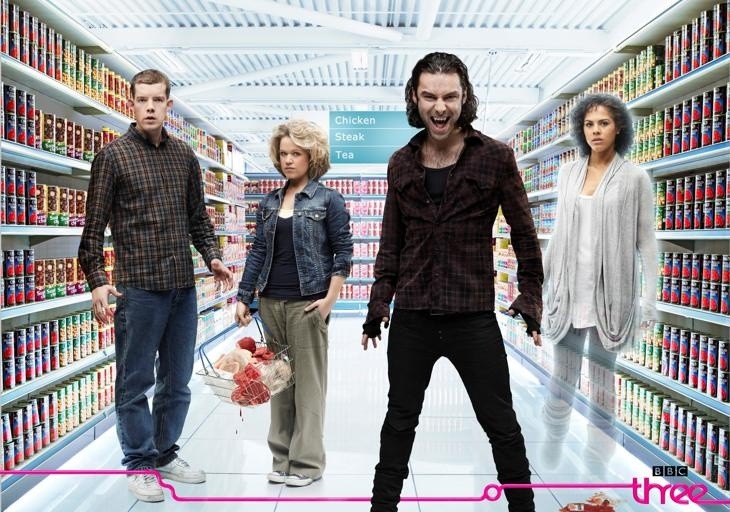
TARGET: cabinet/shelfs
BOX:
[236,169,398,321]
[489,0,729,512]
[0,2,250,512]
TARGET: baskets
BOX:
[196,314,297,408]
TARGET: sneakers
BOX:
[268,468,288,484]
[154,457,207,484]
[126,472,165,503]
[284,475,322,488]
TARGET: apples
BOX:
[238,336,256,354]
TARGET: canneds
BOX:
[0,0,388,477]
[494,0,730,491]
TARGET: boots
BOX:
[540,411,568,470]
[582,425,618,491]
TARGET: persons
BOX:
[77,68,234,504]
[540,95,664,484]
[363,53,545,512]
[236,121,353,487]
